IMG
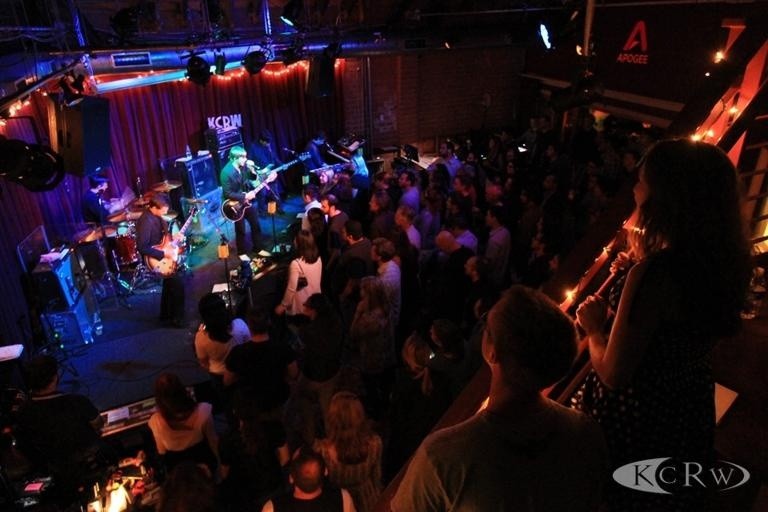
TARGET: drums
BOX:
[79,222,143,271]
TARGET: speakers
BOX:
[175,153,218,199]
[18,246,88,315]
[40,279,102,351]
[46,92,114,179]
[179,186,227,235]
[306,56,336,98]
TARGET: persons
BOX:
[167,1,358,35]
[0,110,675,512]
[570,138,754,511]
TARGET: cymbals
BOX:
[106,180,182,223]
[73,224,117,245]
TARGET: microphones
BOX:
[245,161,260,170]
[192,199,209,203]
[283,147,298,155]
[325,142,334,152]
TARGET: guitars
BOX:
[144,205,200,279]
[222,171,279,222]
[256,152,312,182]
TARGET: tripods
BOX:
[43,314,89,379]
[91,206,136,312]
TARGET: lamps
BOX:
[180,49,305,88]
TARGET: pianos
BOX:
[309,163,351,177]
